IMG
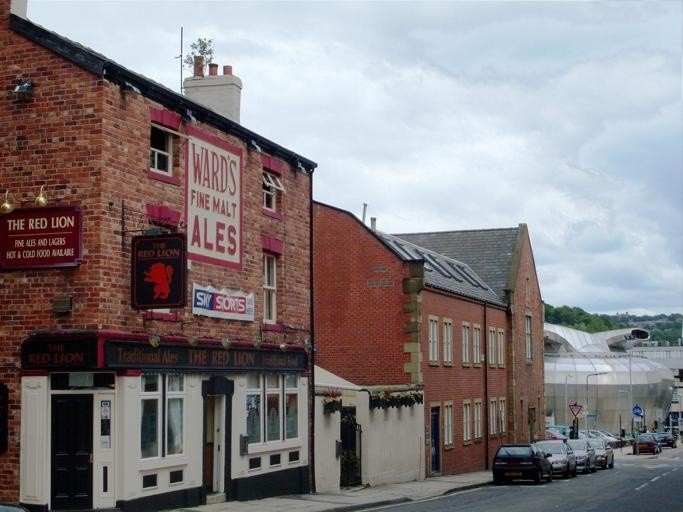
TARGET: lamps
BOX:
[13,80,33,94]
[181,108,197,125]
[34,184,62,208]
[52,294,73,313]
[0,188,25,214]
[294,160,306,175]
[143,318,313,354]
[120,80,142,95]
[246,139,261,154]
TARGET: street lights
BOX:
[583,372,609,440]
[563,373,575,438]
[622,334,639,435]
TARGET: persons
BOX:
[641,424,670,432]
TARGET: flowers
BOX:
[369,386,424,409]
[320,387,343,415]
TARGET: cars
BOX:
[529,439,579,481]
[490,442,554,487]
[541,423,677,475]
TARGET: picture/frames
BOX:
[131,232,188,310]
